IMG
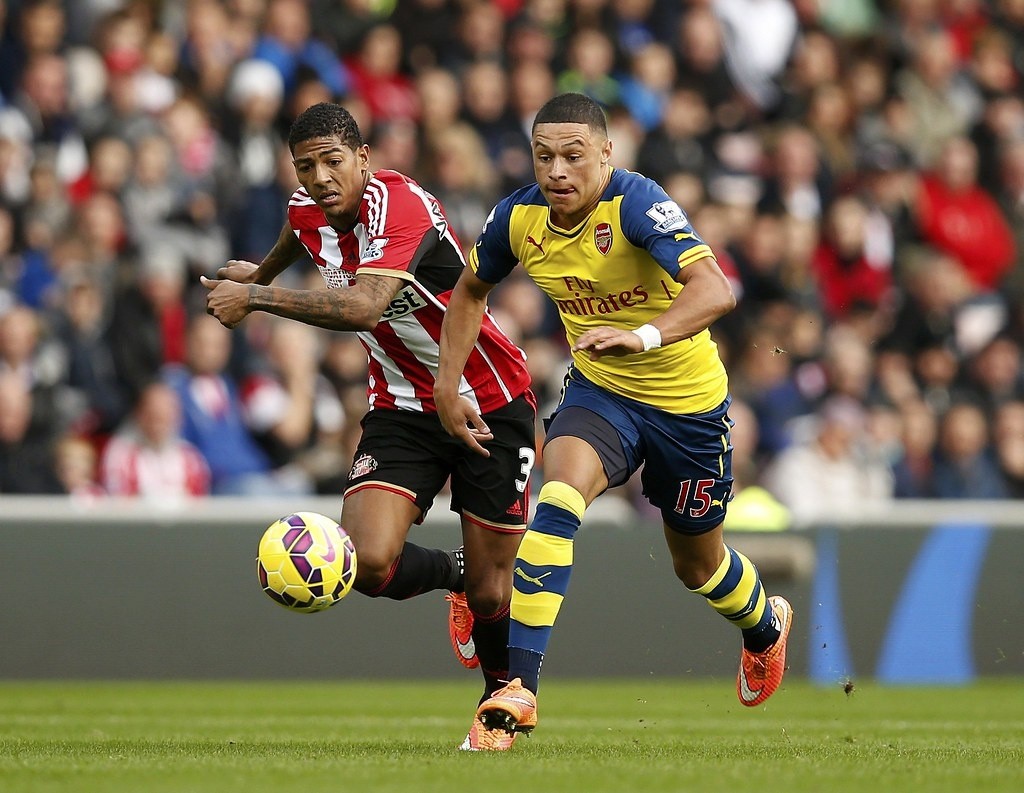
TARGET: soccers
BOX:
[256,513,356,615]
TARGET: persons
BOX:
[198,100,538,751]
[432,92,792,738]
[0,1,1023,503]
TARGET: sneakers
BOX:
[443,545,481,670]
[737,596,793,707]
[459,713,517,751]
[477,678,537,738]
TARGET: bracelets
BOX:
[631,323,662,351]
